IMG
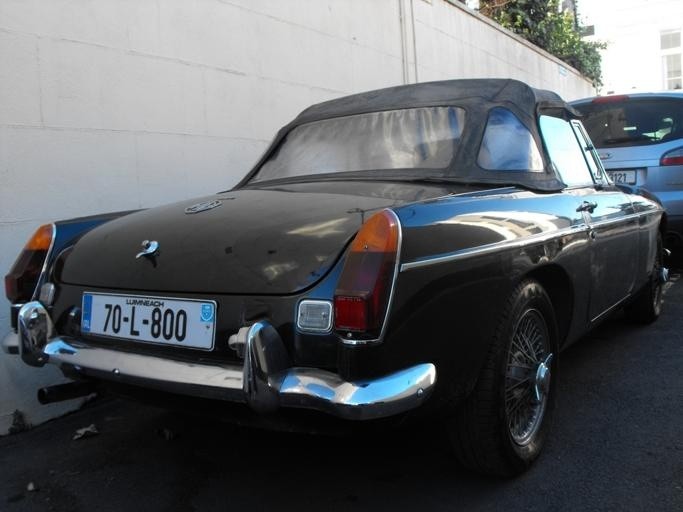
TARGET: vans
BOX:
[561,92,683,267]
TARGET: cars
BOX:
[1,76,667,472]
